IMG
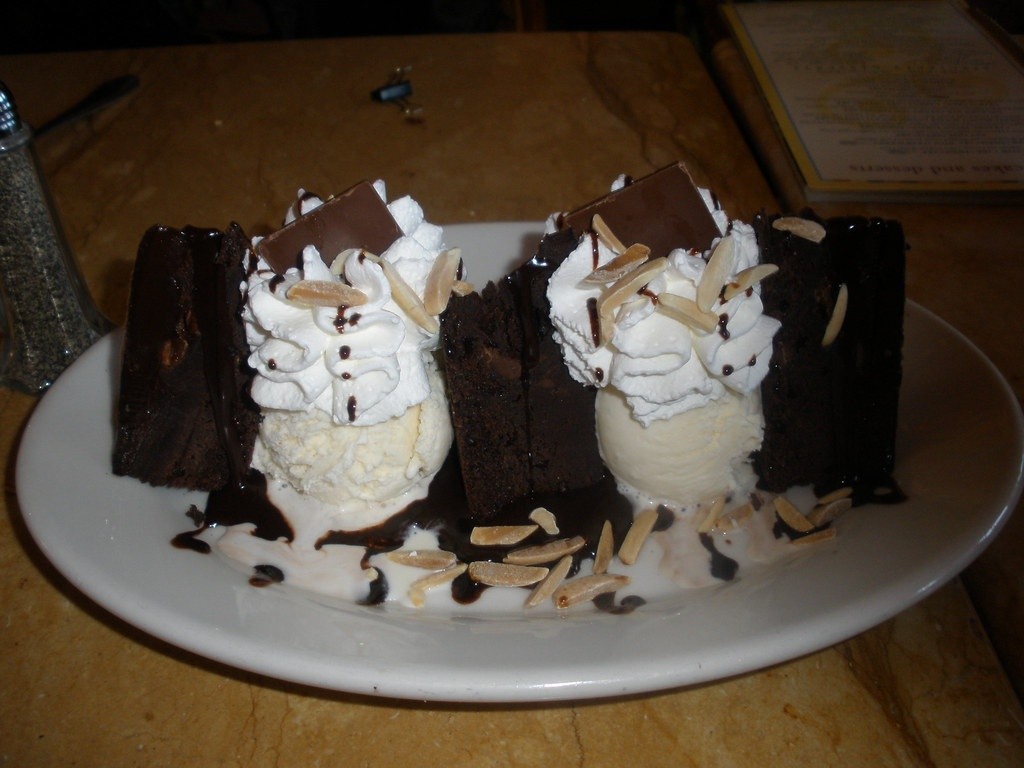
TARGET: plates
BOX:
[18,221,1024,704]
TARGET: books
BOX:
[712,0,1024,205]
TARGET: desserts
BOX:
[110,162,908,611]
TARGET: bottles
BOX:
[0,82,118,396]
